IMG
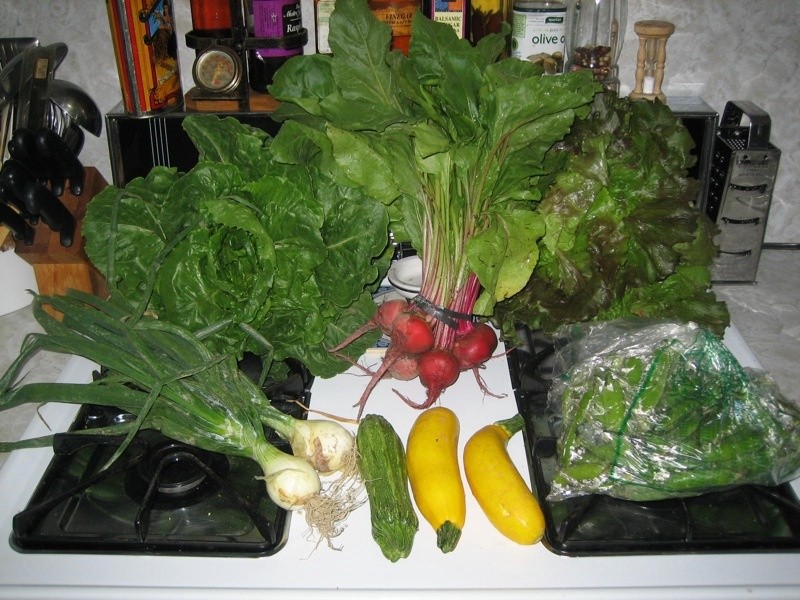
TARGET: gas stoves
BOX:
[4,299,800,599]
[0,272,799,600]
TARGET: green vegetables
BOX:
[75,112,396,377]
[481,87,733,342]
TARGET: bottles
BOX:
[425,0,468,39]
[244,0,304,94]
[367,0,421,57]
[314,0,337,58]
[466,0,568,77]
[189,0,241,40]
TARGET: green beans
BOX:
[547,331,800,502]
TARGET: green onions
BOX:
[0,189,365,543]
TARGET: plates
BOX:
[388,255,422,299]
